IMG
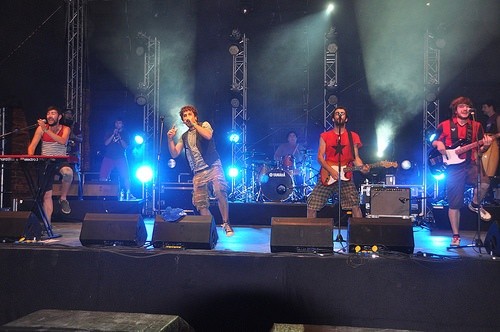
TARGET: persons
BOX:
[100,118,131,180]
[27,106,83,233]
[167,105,234,236]
[273,131,306,187]
[429,97,500,246]
[307,106,370,218]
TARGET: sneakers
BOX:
[41,224,53,235]
[221,222,232,235]
[450,236,461,246]
[468,201,491,221]
[59,198,71,214]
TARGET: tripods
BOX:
[447,112,485,255]
[227,153,265,201]
[294,169,313,202]
[334,116,347,252]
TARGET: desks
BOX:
[0,155,78,240]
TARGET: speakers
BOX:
[79,213,147,247]
[485,219,500,257]
[270,217,334,252]
[0,211,43,241]
[152,214,219,250]
[347,187,414,255]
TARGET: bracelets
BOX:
[192,122,198,126]
[43,124,47,131]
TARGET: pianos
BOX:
[0,153,80,241]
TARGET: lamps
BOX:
[135,85,149,107]
[133,38,147,58]
[401,160,417,175]
[422,83,438,103]
[230,89,243,109]
[324,84,340,106]
[432,35,447,50]
[324,32,339,54]
[228,37,243,56]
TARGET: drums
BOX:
[280,154,297,171]
[261,169,295,202]
[479,132,499,179]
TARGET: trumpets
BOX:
[112,126,121,144]
[68,118,77,148]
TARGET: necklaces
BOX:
[189,127,196,132]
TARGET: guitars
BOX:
[320,158,399,187]
[428,130,500,176]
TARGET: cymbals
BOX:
[239,152,267,156]
[252,160,276,165]
[296,150,319,155]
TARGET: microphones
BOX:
[398,198,405,203]
[278,188,283,193]
[42,119,47,123]
[186,119,190,122]
[467,108,476,113]
[339,113,342,117]
